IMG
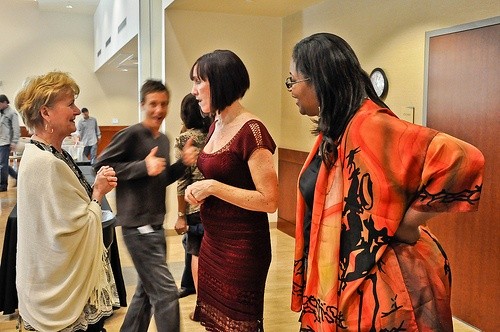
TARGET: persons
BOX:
[285,32,484,331]
[175,92,212,323]
[184,49,280,332]
[92,79,200,332]
[0,94,20,192]
[14,69,122,332]
[176,251,197,300]
[77,108,101,165]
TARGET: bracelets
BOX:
[92,199,103,208]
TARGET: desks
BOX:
[0,165,128,315]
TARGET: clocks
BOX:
[369,68,389,99]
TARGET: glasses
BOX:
[284,76,312,89]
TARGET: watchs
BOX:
[178,212,185,217]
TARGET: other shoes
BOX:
[177,287,196,299]
